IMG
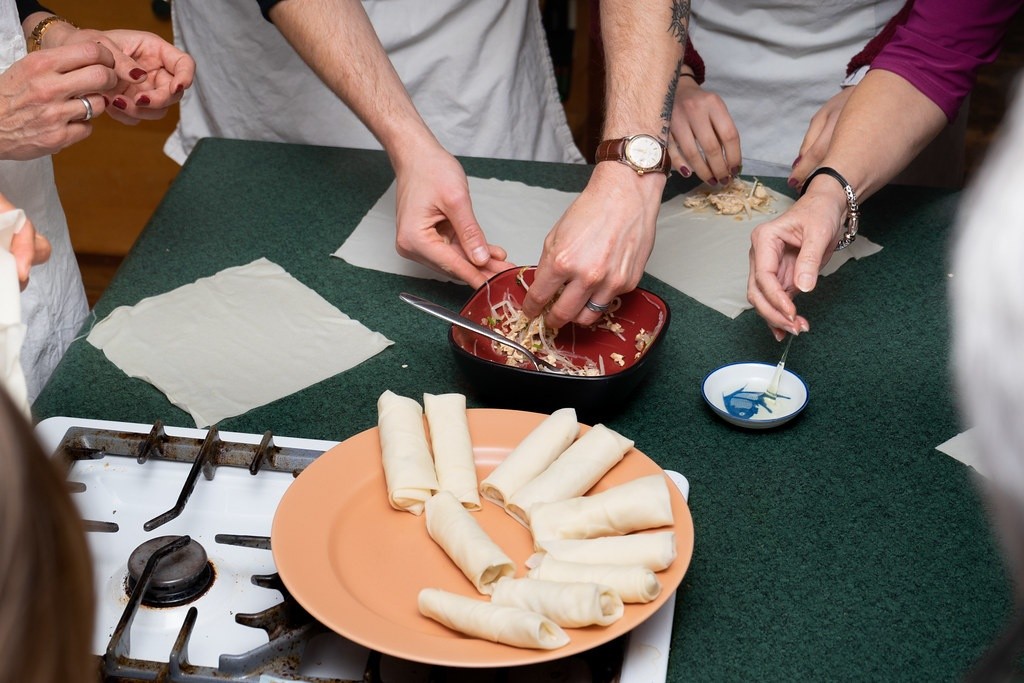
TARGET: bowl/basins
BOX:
[447,265,672,426]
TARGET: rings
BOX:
[586,301,609,310]
[76,96,91,120]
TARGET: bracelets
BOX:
[799,166,859,250]
[26,15,79,53]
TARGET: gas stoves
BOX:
[30,415,444,683]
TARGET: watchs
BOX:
[595,132,671,175]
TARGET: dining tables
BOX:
[28,134,1023,680]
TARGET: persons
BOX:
[744,1,1024,339]
[666,0,966,187]
[0,0,195,408]
[0,389,94,683]
[0,195,50,292]
[148,1,687,329]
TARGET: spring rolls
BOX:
[377,388,676,651]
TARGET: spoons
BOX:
[399,292,579,375]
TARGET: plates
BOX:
[269,407,695,668]
[701,361,809,430]
